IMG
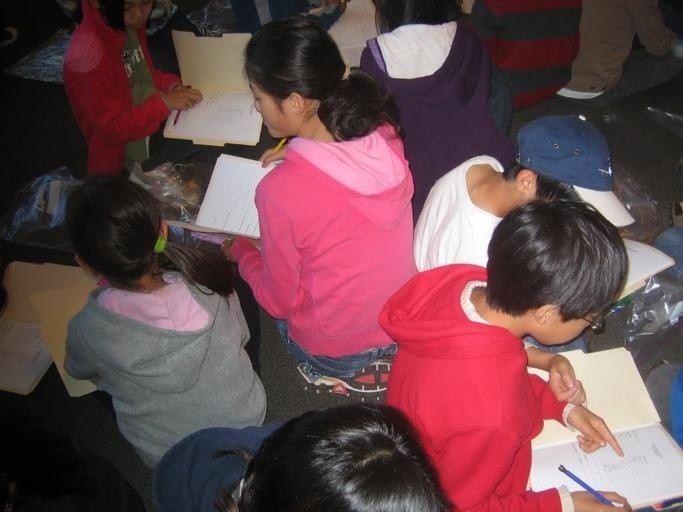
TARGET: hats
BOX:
[514,114,636,229]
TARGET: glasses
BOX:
[556,306,604,334]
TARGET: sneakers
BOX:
[294,357,393,404]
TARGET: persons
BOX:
[62,1,203,177]
[378,197,629,512]
[231,0,682,114]
[644,226,682,446]
[152,402,446,512]
[221,14,416,394]
[61,174,268,470]
[412,115,636,352]
[360,1,518,225]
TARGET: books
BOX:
[617,236,677,300]
[0,261,99,398]
[161,153,280,252]
[164,29,263,148]
[526,347,683,512]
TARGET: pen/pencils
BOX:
[174,85,191,125]
[271,137,289,153]
[558,465,615,507]
